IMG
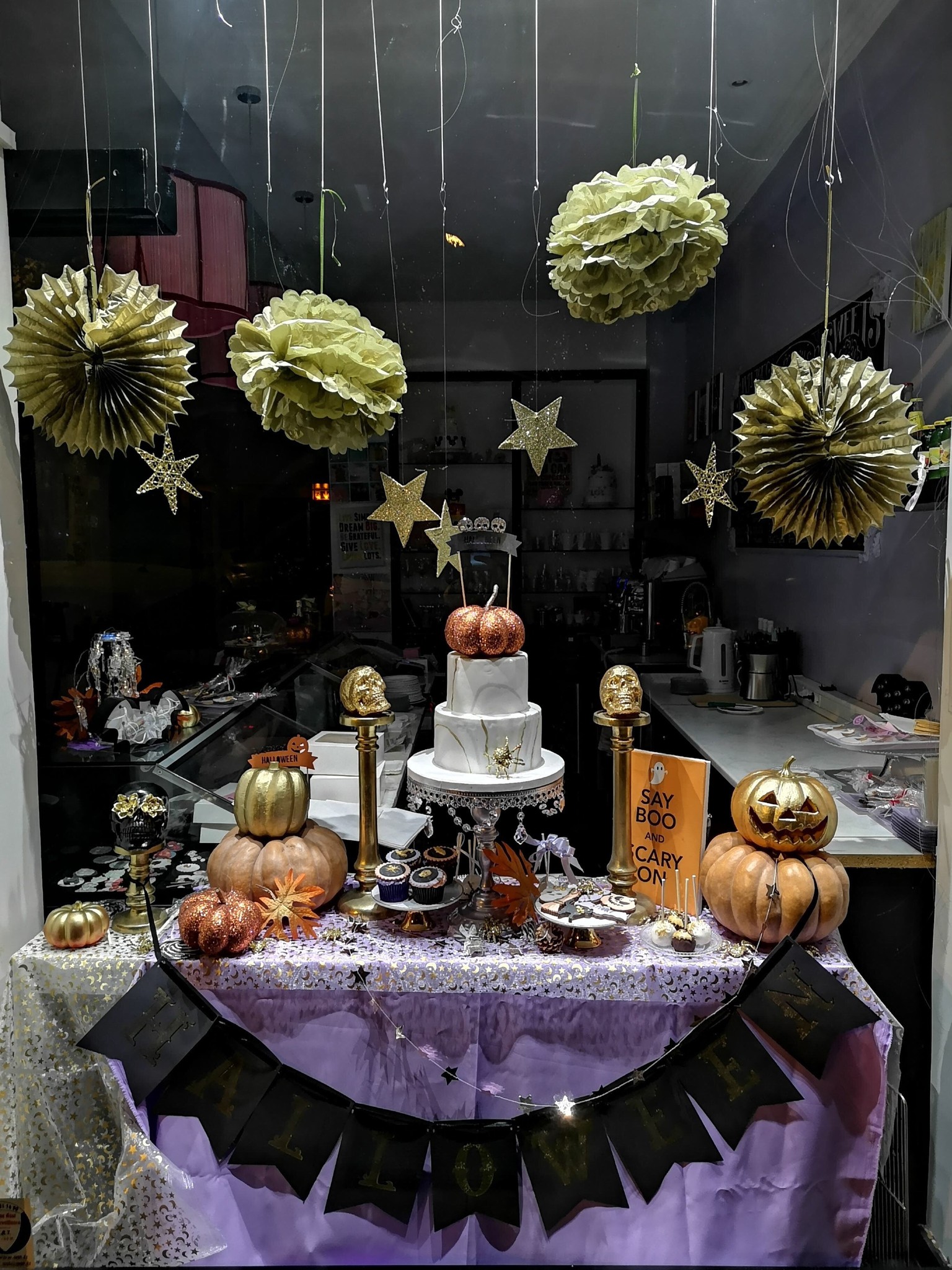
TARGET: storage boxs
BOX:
[299,730,386,807]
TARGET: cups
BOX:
[561,529,630,590]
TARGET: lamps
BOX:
[89,0,291,392]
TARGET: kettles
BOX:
[686,625,738,692]
[741,653,781,701]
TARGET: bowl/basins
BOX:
[816,778,842,796]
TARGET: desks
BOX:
[1,872,904,1270]
[591,636,687,671]
[644,671,940,869]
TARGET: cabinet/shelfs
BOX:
[328,369,648,651]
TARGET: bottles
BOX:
[643,675,652,689]
[758,618,773,633]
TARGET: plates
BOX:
[769,766,825,779]
[717,704,763,715]
[382,675,426,703]
[639,921,722,957]
